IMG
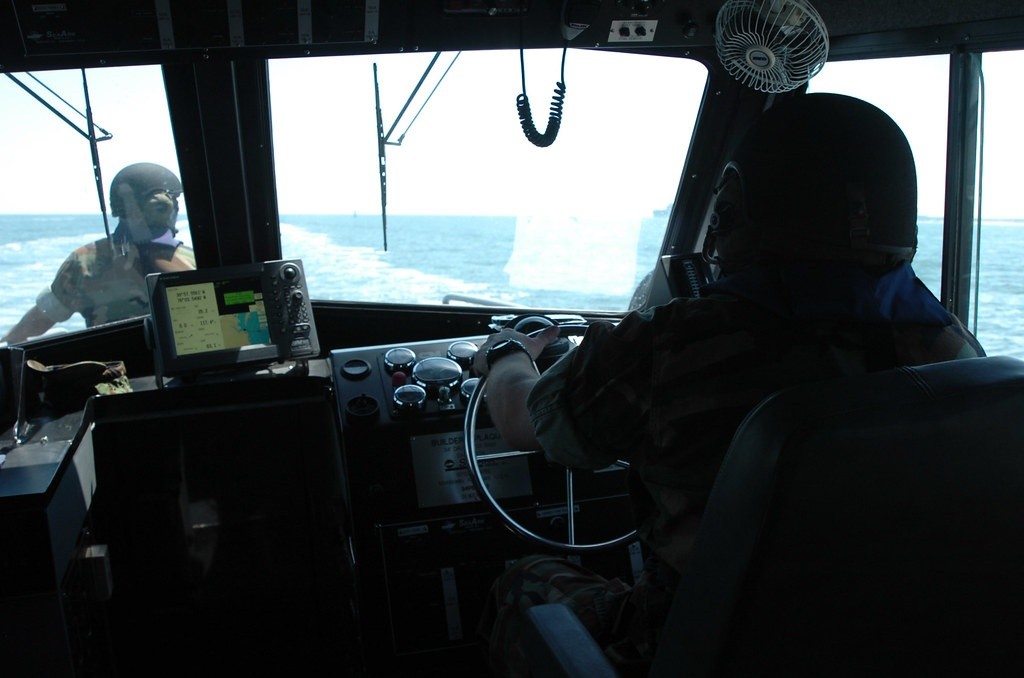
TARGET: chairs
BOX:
[527,358,1024,678]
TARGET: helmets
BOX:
[109,163,183,217]
[710,92,917,262]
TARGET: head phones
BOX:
[144,191,176,227]
[710,170,763,274]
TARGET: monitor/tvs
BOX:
[165,277,273,356]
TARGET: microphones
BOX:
[169,226,179,234]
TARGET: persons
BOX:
[461,92,986,678]
[0,163,198,346]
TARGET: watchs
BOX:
[484,337,533,373]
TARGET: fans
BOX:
[713,2,831,93]
